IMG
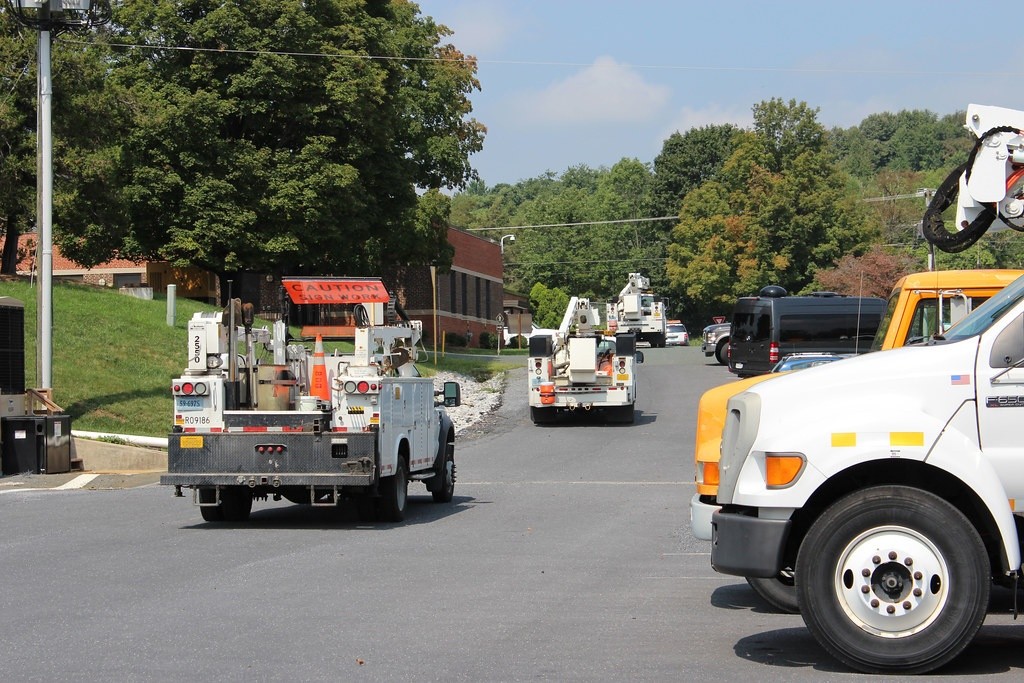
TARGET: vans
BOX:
[727,286,889,378]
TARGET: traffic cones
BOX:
[310,333,329,403]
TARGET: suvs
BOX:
[690,268,1024,613]
[708,96,1024,672]
[702,323,731,366]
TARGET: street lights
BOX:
[496,314,506,356]
[914,186,940,270]
[4,0,107,426]
[501,234,516,256]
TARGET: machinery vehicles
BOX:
[165,275,462,522]
[528,295,644,426]
[607,270,666,347]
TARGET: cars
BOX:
[771,350,844,373]
[666,320,688,344]
[774,358,846,372]
